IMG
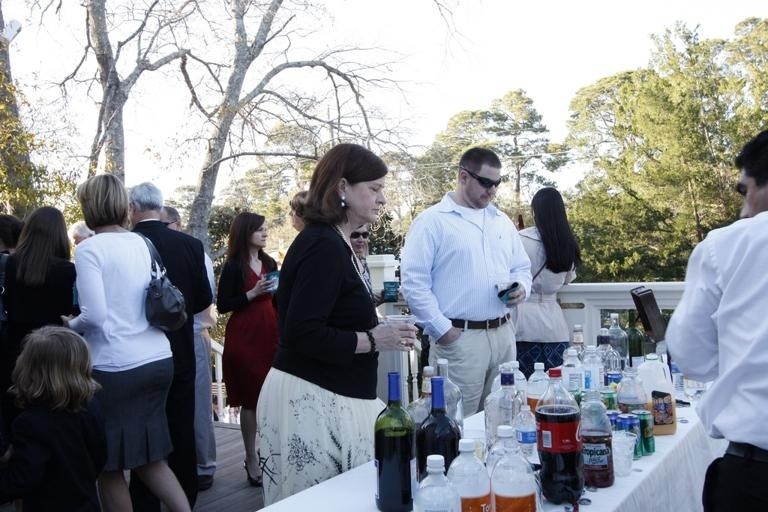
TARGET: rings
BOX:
[400,338,407,347]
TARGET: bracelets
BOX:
[364,330,376,355]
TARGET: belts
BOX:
[449,313,510,329]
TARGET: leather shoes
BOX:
[197,475,213,491]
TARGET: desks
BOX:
[246,381,728,512]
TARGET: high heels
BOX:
[243,452,263,487]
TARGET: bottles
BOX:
[436,358,466,436]
[483,373,527,452]
[615,413,643,460]
[485,424,513,476]
[535,368,585,505]
[606,410,622,430]
[416,378,460,482]
[632,409,655,456]
[617,366,647,413]
[407,366,436,422]
[562,350,581,390]
[608,313,629,370]
[670,358,683,394]
[563,325,586,363]
[490,360,527,394]
[580,346,604,388]
[683,379,706,396]
[446,439,491,512]
[413,454,461,512]
[490,440,537,512]
[606,371,621,386]
[527,362,549,413]
[624,309,644,368]
[600,390,617,410]
[513,404,536,457]
[579,390,614,488]
[373,371,418,512]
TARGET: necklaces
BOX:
[336,224,372,298]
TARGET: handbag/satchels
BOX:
[136,231,187,333]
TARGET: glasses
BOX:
[351,231,369,238]
[460,166,501,188]
[163,220,177,226]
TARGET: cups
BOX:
[613,430,637,476]
[386,315,415,352]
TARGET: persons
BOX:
[0,213,24,324]
[68,220,95,246]
[663,130,768,511]
[350,225,400,325]
[61,174,193,511]
[255,144,418,508]
[5,207,77,327]
[217,213,278,485]
[288,191,306,232]
[517,187,582,382]
[128,182,213,512]
[164,206,218,492]
[8,325,109,512]
[400,148,533,422]
